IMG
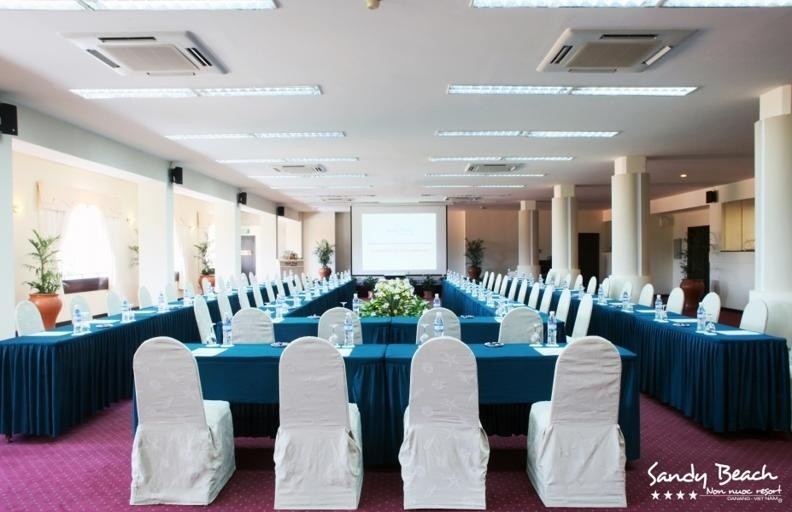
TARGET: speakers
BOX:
[170,166,183,185]
[0,104,18,135]
[238,191,248,205]
[277,206,284,216]
[705,190,717,203]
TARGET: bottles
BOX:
[220,309,559,349]
[507,268,707,332]
[433,294,442,308]
[275,269,351,318]
[352,293,359,319]
[70,269,294,335]
[445,269,509,316]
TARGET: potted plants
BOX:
[312,238,338,280]
[679,229,722,307]
[463,237,485,278]
[27,230,64,329]
[190,232,215,293]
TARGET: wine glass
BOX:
[79,304,135,333]
[206,322,544,346]
[627,297,716,334]
